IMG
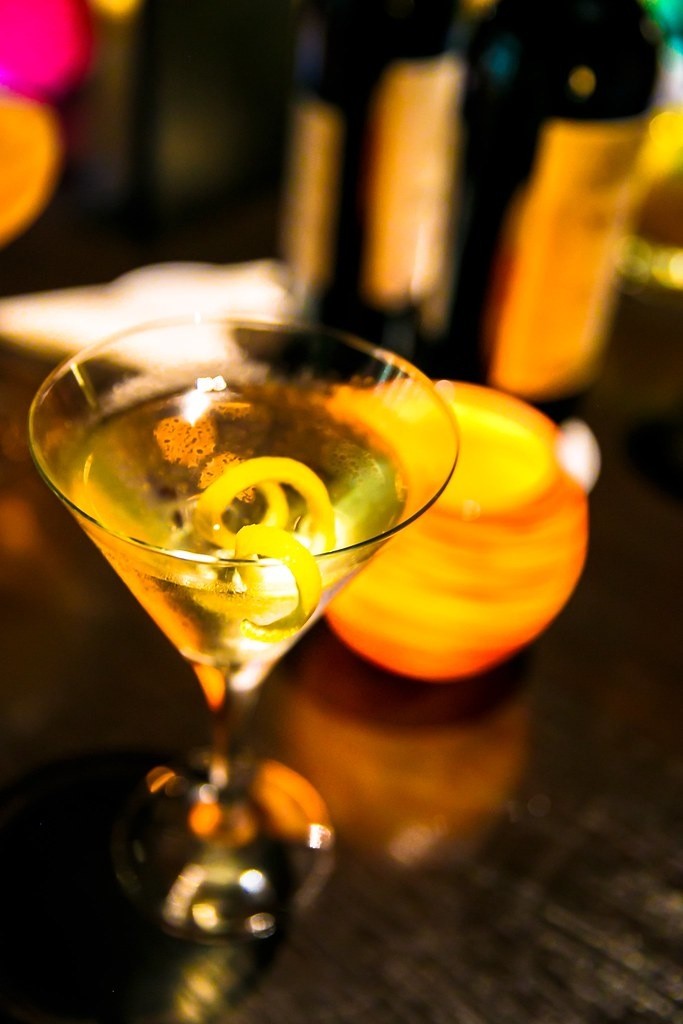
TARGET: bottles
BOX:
[284,0,457,361]
[434,0,665,406]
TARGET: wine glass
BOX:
[27,317,454,950]
